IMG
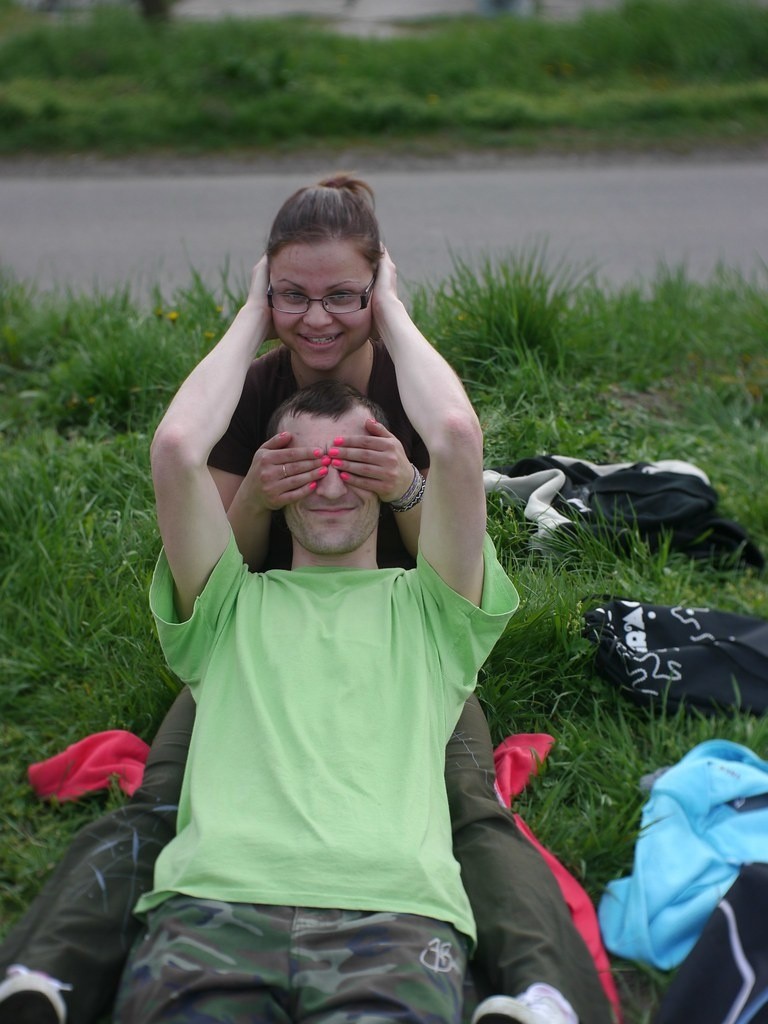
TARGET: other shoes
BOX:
[470,982,580,1024]
[0,963,73,1024]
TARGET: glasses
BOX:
[268,277,376,314]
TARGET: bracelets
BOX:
[390,463,426,512]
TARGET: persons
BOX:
[113,241,521,1024]
[0,175,618,1023]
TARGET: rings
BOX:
[283,464,288,477]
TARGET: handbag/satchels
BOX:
[579,593,768,721]
